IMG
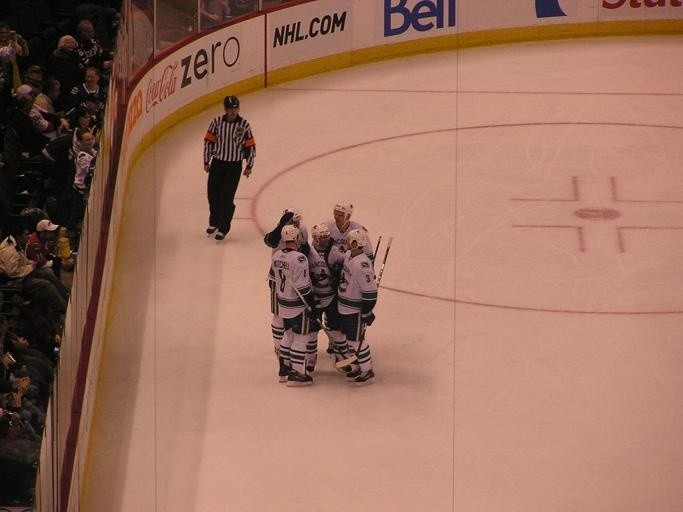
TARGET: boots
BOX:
[344,365,375,384]
[327,344,334,353]
[206,225,230,240]
[279,357,316,382]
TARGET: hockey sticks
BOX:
[335,237,392,368]
[281,267,359,365]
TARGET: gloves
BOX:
[307,309,323,319]
[277,212,294,227]
[362,311,375,325]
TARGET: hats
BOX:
[36,219,60,232]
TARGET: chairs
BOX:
[0,1,105,507]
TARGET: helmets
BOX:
[224,96,239,108]
[281,201,367,247]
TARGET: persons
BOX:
[0,4,122,465]
[200,0,232,30]
[203,95,257,240]
[263,201,379,387]
